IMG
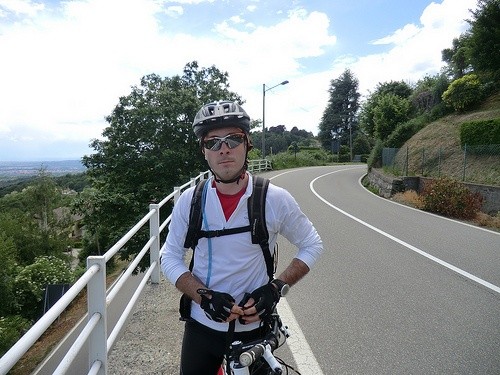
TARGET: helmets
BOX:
[191,101,251,136]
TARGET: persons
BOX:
[160,100,324,375]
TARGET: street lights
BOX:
[262,80,288,159]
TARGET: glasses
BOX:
[202,133,247,152]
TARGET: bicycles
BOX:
[217,312,302,375]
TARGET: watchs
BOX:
[270,279,289,297]
